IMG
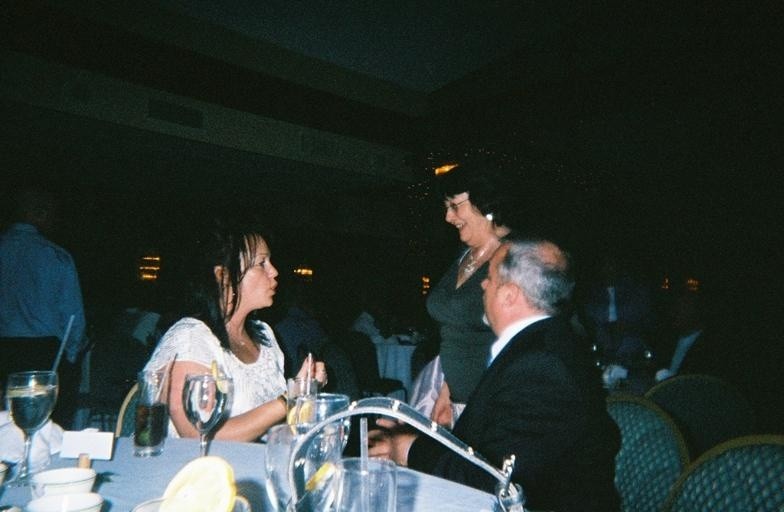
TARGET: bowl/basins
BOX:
[130,494,252,512]
[30,467,96,498]
[27,492,104,512]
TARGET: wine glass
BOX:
[181,371,230,458]
[3,370,60,489]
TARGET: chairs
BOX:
[114,382,140,437]
[605,374,784,512]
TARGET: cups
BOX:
[262,375,399,512]
[132,367,171,459]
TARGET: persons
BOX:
[369,163,623,512]
[144,219,327,441]
[2,186,87,431]
[277,277,328,378]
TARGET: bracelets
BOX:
[279,394,292,411]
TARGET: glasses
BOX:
[444,198,468,213]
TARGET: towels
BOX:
[0,410,65,468]
